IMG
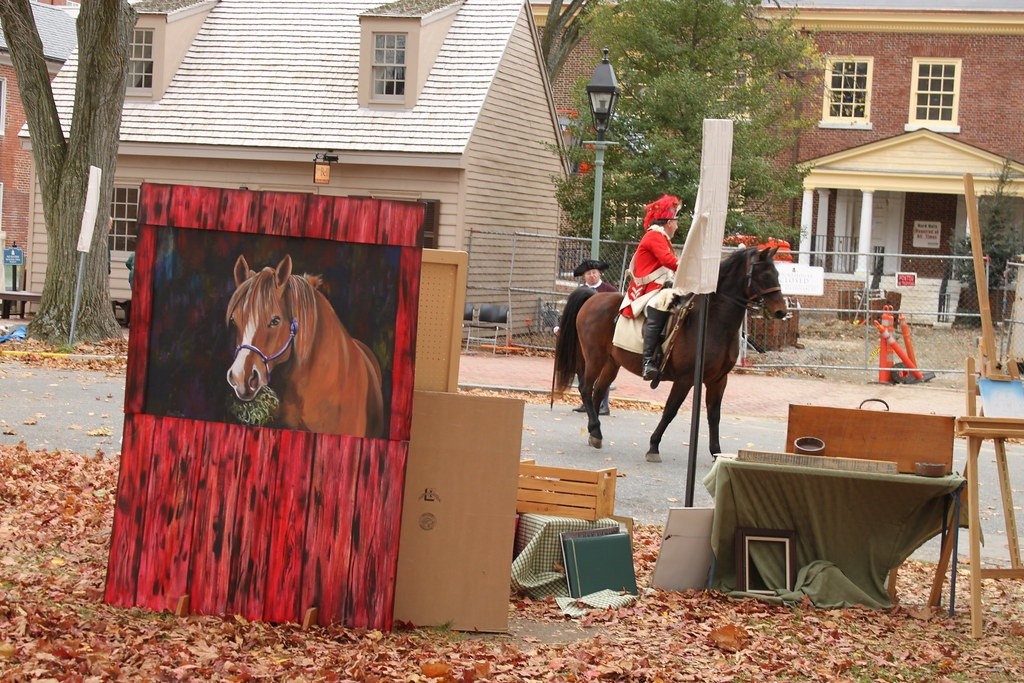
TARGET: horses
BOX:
[554,245,788,463]
[225,254,385,438]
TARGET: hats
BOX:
[643,196,683,227]
[574,257,610,277]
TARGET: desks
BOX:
[707,454,966,618]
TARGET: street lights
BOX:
[582,48,620,260]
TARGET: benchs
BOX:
[0,290,130,329]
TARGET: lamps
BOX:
[311,151,341,184]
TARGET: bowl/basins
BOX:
[915,461,946,477]
[794,436,826,456]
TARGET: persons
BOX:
[554,260,617,416]
[618,196,683,380]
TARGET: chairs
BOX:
[461,300,509,356]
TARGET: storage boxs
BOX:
[787,403,955,475]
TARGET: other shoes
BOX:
[598,406,610,415]
[572,403,586,412]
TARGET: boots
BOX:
[641,306,669,380]
